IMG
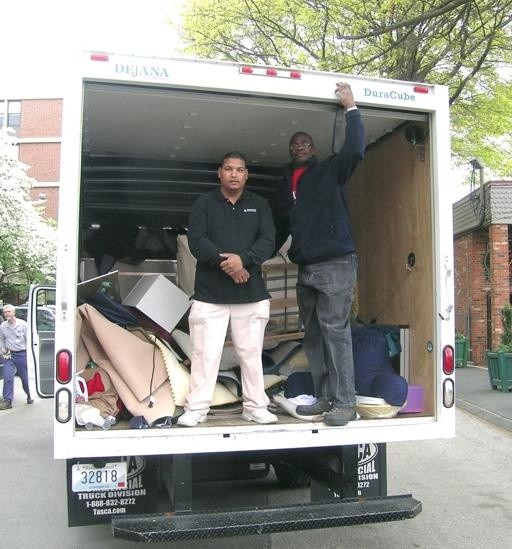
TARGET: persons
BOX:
[176,152,279,428]
[272,81,367,425]
[0,304,33,411]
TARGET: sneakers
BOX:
[323,404,358,427]
[239,406,279,426]
[175,409,209,428]
[294,398,334,417]
[0,399,13,410]
[27,397,34,404]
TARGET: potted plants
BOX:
[485,305,512,392]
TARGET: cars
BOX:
[0,305,55,379]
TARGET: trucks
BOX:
[28,48,457,545]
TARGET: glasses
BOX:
[289,143,313,149]
